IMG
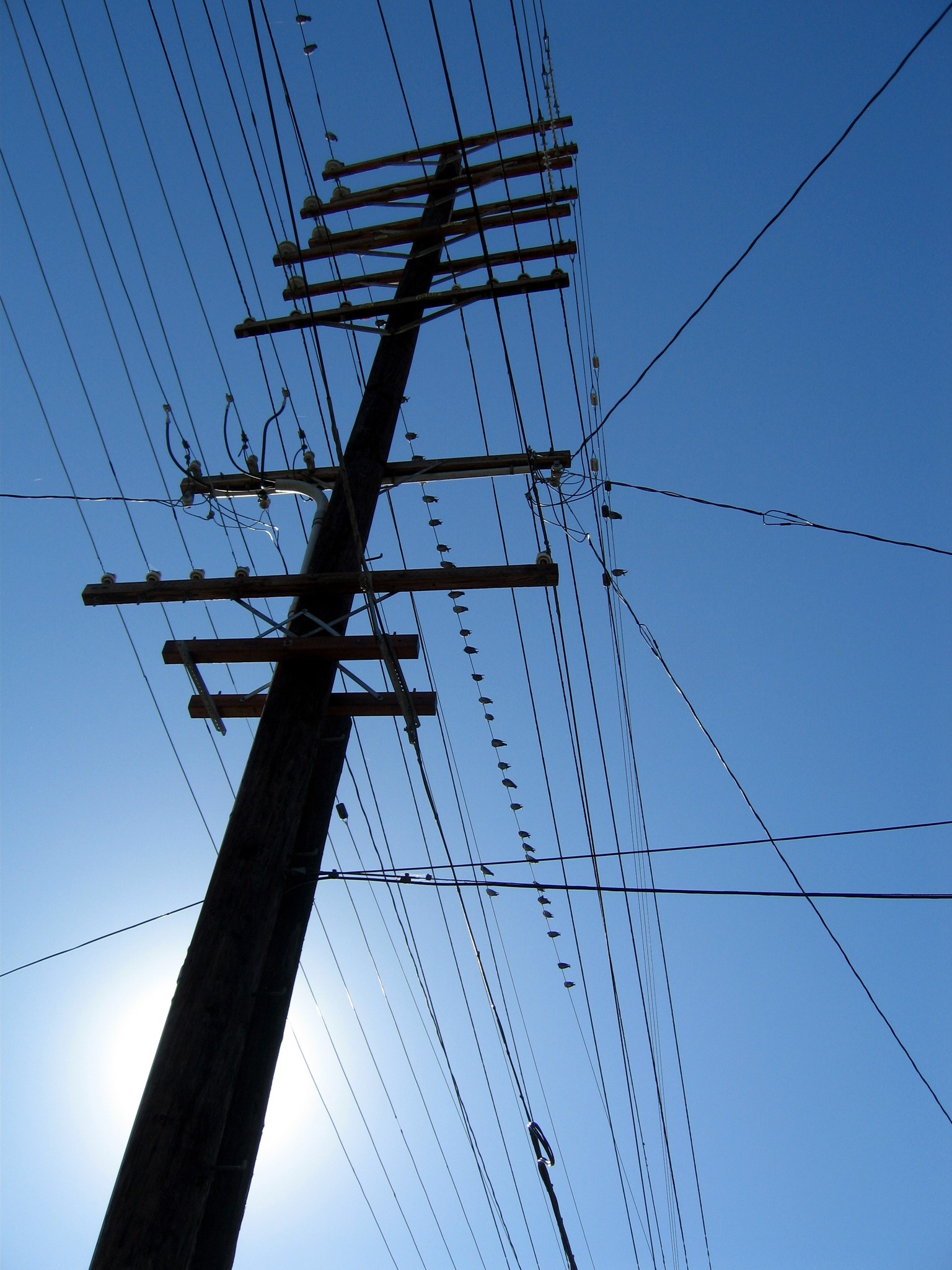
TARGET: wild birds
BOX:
[452,606,469,613]
[411,455,427,461]
[422,494,439,503]
[478,696,577,988]
[479,865,495,877]
[375,319,387,327]
[471,674,486,681]
[296,14,312,25]
[428,519,443,527]
[612,569,628,578]
[440,561,456,568]
[608,511,624,520]
[325,131,338,142]
[448,591,466,599]
[463,646,479,655]
[459,629,472,636]
[486,888,499,897]
[401,395,411,404]
[405,432,419,440]
[436,544,452,553]
[302,43,318,56]
